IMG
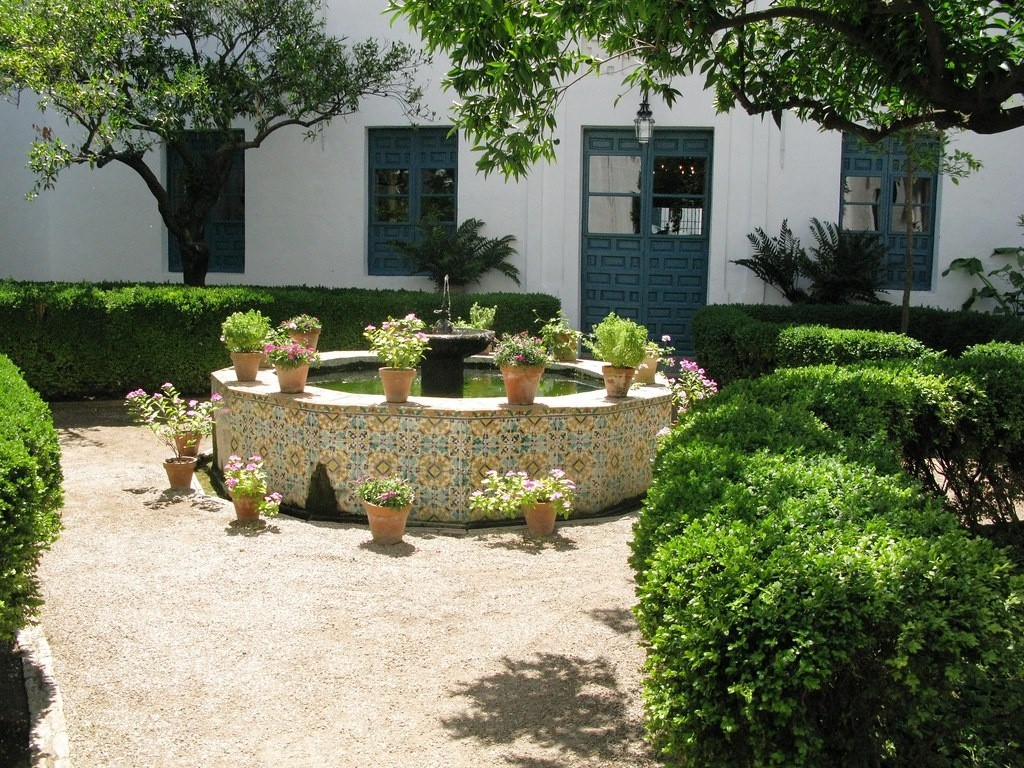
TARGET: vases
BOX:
[173,434,203,455]
[362,499,413,545]
[275,364,310,393]
[500,364,545,405]
[521,503,558,535]
[379,367,416,403]
[634,351,660,384]
[288,329,321,350]
[164,457,197,491]
[230,491,264,522]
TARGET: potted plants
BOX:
[581,312,649,397]
[453,302,498,355]
[220,309,278,382]
[531,308,583,362]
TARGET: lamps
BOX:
[633,69,656,144]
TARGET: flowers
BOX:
[264,338,323,376]
[355,474,415,511]
[223,456,283,520]
[656,359,718,436]
[123,383,229,463]
[468,468,576,520]
[637,336,677,369]
[276,314,319,338]
[363,313,432,372]
[489,330,553,369]
